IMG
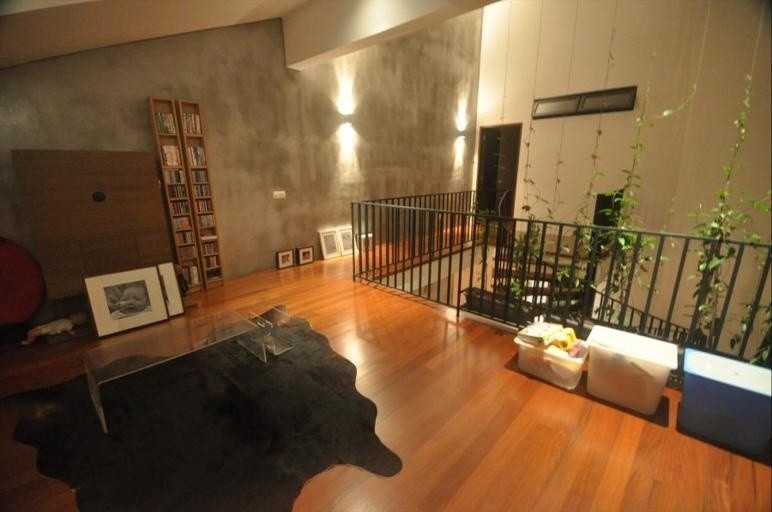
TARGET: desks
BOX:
[78,305,292,435]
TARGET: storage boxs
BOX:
[513,313,770,466]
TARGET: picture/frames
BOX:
[84,262,186,339]
[275,243,316,273]
[318,224,373,260]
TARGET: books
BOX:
[154,110,221,288]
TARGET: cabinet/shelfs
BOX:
[148,91,225,298]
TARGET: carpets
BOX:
[0,300,405,511]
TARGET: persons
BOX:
[109,284,150,320]
